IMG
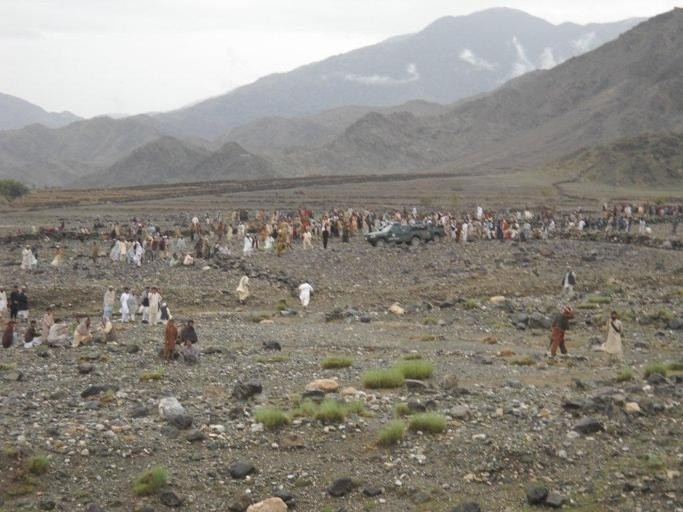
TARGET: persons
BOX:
[163,317,177,359]
[560,267,576,299]
[236,273,250,305]
[298,281,314,308]
[180,339,199,365]
[606,311,623,362]
[545,304,574,359]
[180,319,198,343]
[0,284,173,348]
[16,199,682,271]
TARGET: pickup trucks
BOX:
[366,222,443,247]
